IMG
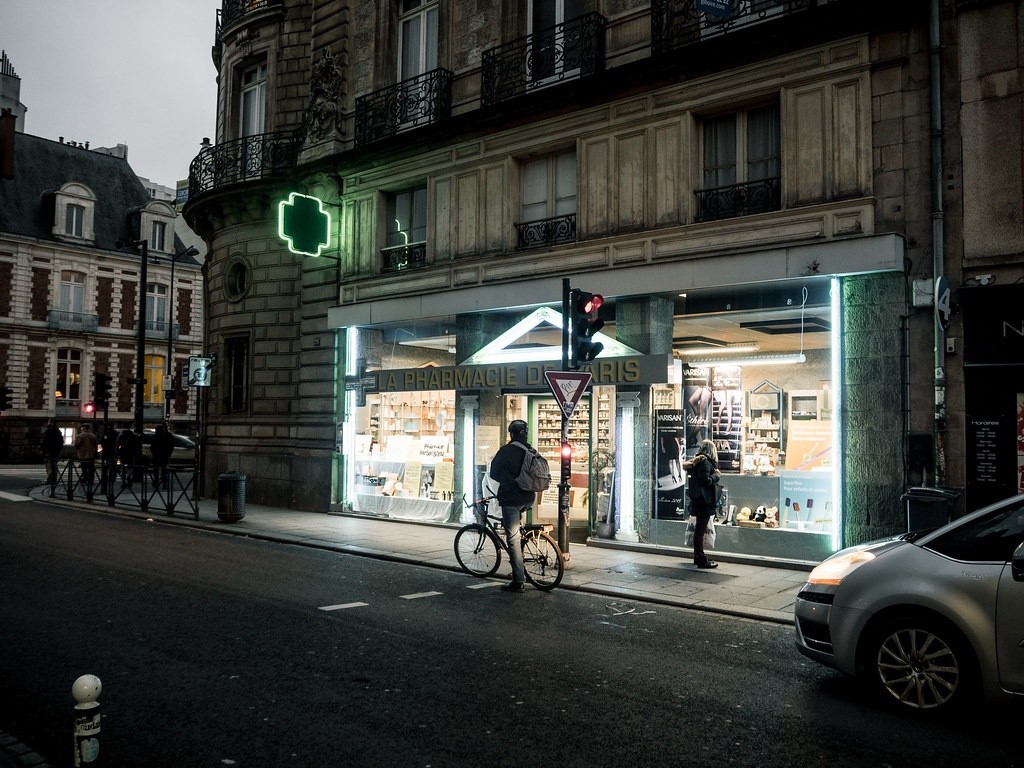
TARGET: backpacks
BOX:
[510,441,552,492]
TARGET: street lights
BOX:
[115,235,148,429]
[149,245,200,428]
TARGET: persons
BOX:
[716,389,733,435]
[75,423,98,485]
[118,424,137,488]
[490,420,552,591]
[101,422,117,484]
[39,418,64,484]
[686,386,712,447]
[682,439,722,569]
[150,423,174,488]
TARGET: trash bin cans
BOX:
[216,471,246,522]
[900,487,961,534]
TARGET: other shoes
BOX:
[707,561,714,564]
[500,582,525,593]
[698,563,718,568]
[121,483,126,488]
[128,479,133,488]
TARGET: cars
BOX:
[111,427,201,470]
[794,490,1024,723]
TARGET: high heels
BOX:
[687,439,697,448]
[724,428,733,436]
[672,476,677,484]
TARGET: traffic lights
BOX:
[561,444,572,480]
[571,289,605,366]
[84,402,95,413]
[95,373,113,403]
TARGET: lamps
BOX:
[688,286,808,368]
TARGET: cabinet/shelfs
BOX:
[533,403,611,463]
[368,401,456,438]
[713,400,742,465]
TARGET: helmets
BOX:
[509,420,529,433]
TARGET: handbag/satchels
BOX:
[684,515,716,548]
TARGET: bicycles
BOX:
[454,484,566,591]
